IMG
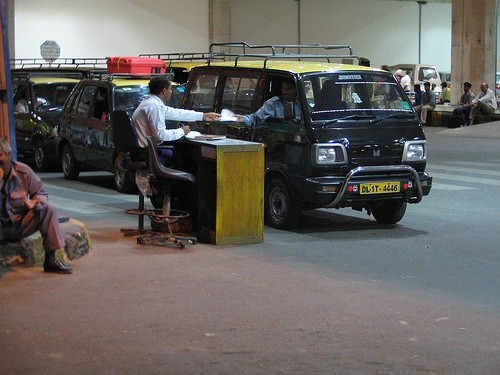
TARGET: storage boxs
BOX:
[106,56,167,79]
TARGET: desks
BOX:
[182,133,266,245]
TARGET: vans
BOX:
[382,64,442,103]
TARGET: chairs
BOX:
[316,80,346,109]
[109,107,178,235]
[136,136,201,250]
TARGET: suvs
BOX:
[9,53,257,194]
[173,42,433,230]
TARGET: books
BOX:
[184,131,226,140]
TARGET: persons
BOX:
[469,83,497,126]
[101,93,129,122]
[234,80,314,127]
[414,82,436,127]
[449,82,476,128]
[436,81,450,105]
[16,89,48,113]
[412,84,424,106]
[395,69,412,95]
[131,77,223,176]
[0,139,72,275]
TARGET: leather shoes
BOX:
[43,257,72,273]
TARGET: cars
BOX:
[496,72,500,102]
[426,73,451,88]
